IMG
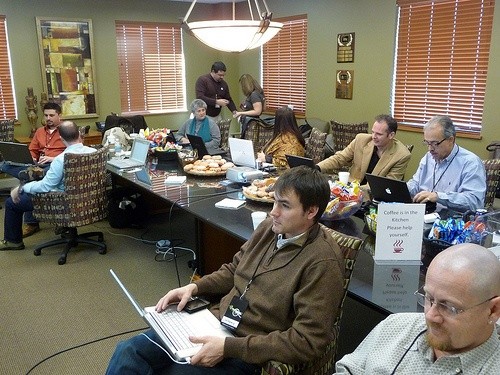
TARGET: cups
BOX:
[251,212,267,231]
[339,172,350,185]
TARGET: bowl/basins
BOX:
[317,197,363,220]
[364,213,376,236]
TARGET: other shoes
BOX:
[22,223,40,237]
[0,239,25,251]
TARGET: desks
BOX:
[107,156,500,351]
[13,130,102,145]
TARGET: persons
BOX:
[257,107,305,169]
[195,61,238,122]
[23,103,67,238]
[0,120,97,250]
[316,114,412,190]
[233,73,265,138]
[406,115,487,214]
[104,165,345,375]
[174,99,221,152]
[333,242,500,375]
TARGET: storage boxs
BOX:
[422,227,494,267]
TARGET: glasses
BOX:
[424,138,446,148]
[414,284,498,317]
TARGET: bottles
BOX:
[115,139,122,159]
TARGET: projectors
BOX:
[227,166,264,184]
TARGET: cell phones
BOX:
[183,298,211,313]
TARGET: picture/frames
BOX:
[34,15,100,121]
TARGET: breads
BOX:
[184,155,235,174]
[242,177,278,199]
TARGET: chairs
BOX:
[256,223,366,375]
[101,115,148,139]
[26,145,108,264]
[241,116,274,155]
[329,119,369,152]
[216,118,232,158]
[481,158,500,207]
[305,127,328,162]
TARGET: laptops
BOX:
[107,138,150,169]
[229,138,261,168]
[285,153,316,170]
[0,141,41,167]
[109,269,234,362]
[366,172,414,205]
[170,130,190,145]
[186,133,227,155]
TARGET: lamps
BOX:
[178,0,284,55]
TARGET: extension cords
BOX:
[157,240,171,246]
